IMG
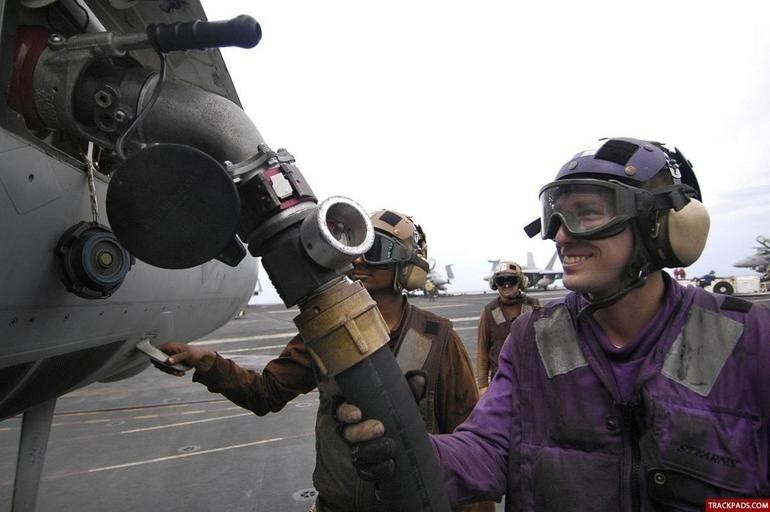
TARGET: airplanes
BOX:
[483,251,564,294]
[403,258,454,297]
[0,1,275,511]
[733,235,770,279]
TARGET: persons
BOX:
[698,270,717,287]
[425,279,436,303]
[476,258,543,399]
[150,204,496,512]
[335,138,770,511]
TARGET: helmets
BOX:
[555,136,709,276]
[371,209,431,295]
[488,260,528,288]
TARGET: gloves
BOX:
[331,371,428,482]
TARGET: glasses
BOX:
[540,178,650,238]
[495,274,519,288]
[362,231,401,264]
